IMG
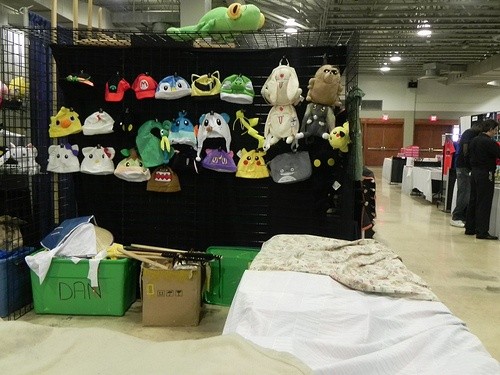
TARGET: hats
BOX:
[190,71,220,97]
[168,112,197,152]
[113,149,150,182]
[48,106,82,138]
[221,75,254,103]
[47,145,79,174]
[80,145,115,175]
[135,120,175,167]
[8,77,29,99]
[132,73,157,100]
[155,75,192,99]
[236,148,270,178]
[105,75,130,102]
[83,108,113,136]
[201,148,236,173]
[147,166,181,193]
[113,110,137,137]
[196,112,235,162]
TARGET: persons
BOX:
[449,120,483,228]
[465,119,500,240]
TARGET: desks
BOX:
[403,167,445,202]
[382,157,441,183]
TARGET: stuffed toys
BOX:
[166,3,265,45]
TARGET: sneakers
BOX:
[450,219,465,228]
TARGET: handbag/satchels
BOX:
[263,104,300,152]
[260,57,304,106]
[0,215,23,251]
[296,104,335,139]
[267,151,312,183]
[306,64,343,106]
[330,125,351,152]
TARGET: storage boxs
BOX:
[26,248,136,317]
[139,255,206,327]
[0,247,35,318]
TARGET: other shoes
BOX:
[465,229,477,235]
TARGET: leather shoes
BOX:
[476,232,497,240]
